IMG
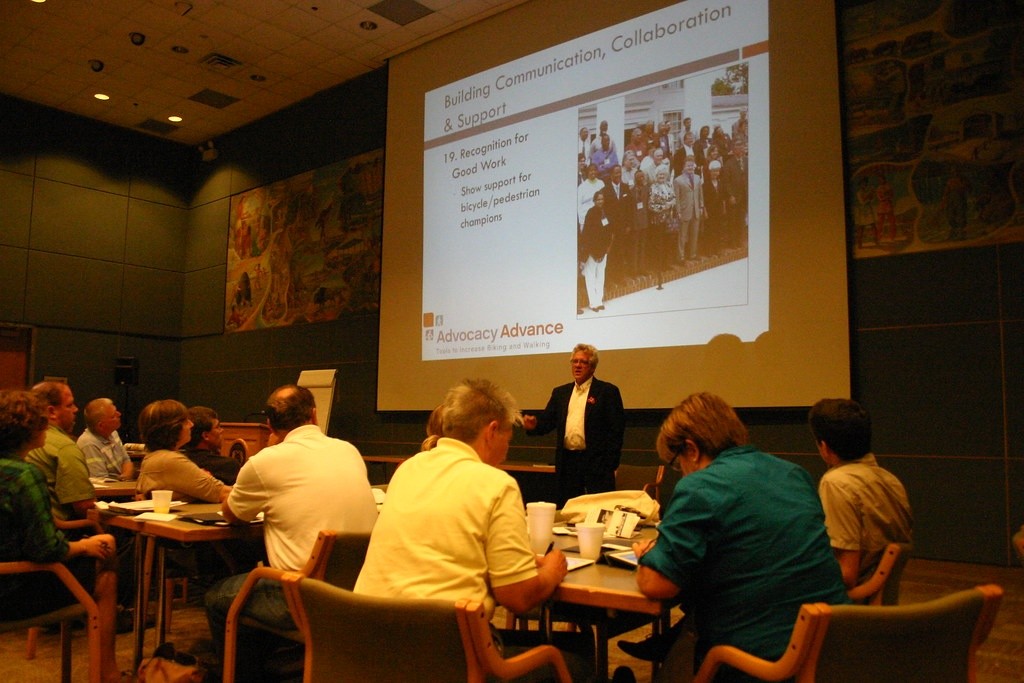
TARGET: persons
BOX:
[182,406,240,486]
[353,378,636,683]
[0,388,136,683]
[637,391,855,683]
[138,400,258,572]
[24,379,159,634]
[618,398,912,662]
[522,344,625,509]
[76,398,134,481]
[578,106,748,315]
[201,384,370,683]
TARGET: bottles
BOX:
[123,442,152,452]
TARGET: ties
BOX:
[688,173,694,189]
[582,140,585,158]
[615,186,619,195]
[737,159,743,170]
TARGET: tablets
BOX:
[565,558,596,572]
[608,550,638,570]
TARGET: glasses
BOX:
[669,452,681,472]
[570,358,591,365]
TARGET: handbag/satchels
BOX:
[138,642,209,683]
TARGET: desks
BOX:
[92,480,139,496]
[551,519,680,683]
[125,450,148,470]
[87,502,263,674]
[363,455,556,493]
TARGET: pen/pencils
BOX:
[545,541,555,556]
[84,534,113,550]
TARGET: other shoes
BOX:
[115,609,157,633]
[592,308,599,312]
[617,631,674,663]
[612,666,637,683]
[599,305,604,310]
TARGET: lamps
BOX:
[197,140,219,160]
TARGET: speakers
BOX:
[115,357,139,385]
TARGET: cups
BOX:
[151,490,173,515]
[576,522,605,562]
[527,501,557,556]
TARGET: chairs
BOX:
[1,464,1004,683]
